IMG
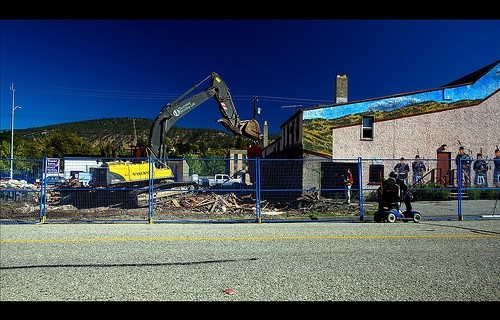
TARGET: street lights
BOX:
[10,105,22,179]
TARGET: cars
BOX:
[40,176,66,187]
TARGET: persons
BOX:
[493,149,500,184]
[386,171,397,190]
[456,146,471,182]
[412,155,426,184]
[343,168,353,204]
[394,158,410,184]
[473,153,488,186]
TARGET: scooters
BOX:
[373,184,422,223]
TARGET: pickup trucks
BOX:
[198,173,241,188]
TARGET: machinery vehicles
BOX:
[60,71,263,209]
